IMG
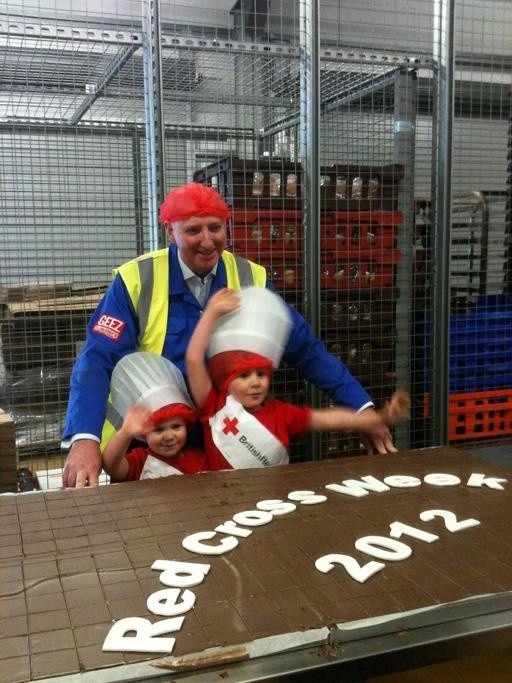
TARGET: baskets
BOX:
[194,153,511,443]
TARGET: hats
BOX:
[209,350,273,392]
[158,181,233,226]
[207,286,295,370]
[107,350,196,443]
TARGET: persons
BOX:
[53,181,402,493]
[96,394,208,486]
[184,286,419,476]
